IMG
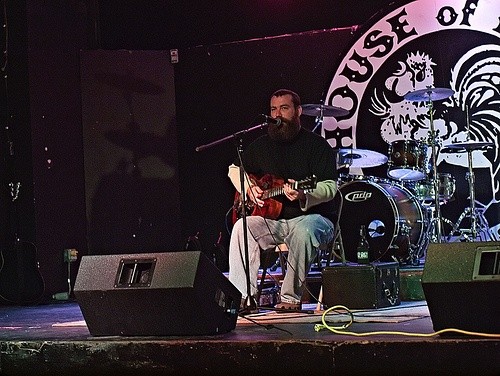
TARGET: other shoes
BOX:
[275,302,302,313]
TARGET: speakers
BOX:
[73,251,243,337]
[420,240,500,338]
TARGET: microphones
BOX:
[260,113,283,128]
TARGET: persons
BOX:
[227,89,337,313]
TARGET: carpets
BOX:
[52,300,432,326]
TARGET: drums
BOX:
[328,180,425,265]
[386,139,427,182]
[415,172,455,200]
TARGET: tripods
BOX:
[195,123,315,314]
[416,98,496,258]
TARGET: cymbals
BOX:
[404,87,455,102]
[301,103,350,118]
[333,149,389,168]
[443,142,495,149]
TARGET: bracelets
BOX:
[247,185,254,196]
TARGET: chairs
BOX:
[257,188,345,311]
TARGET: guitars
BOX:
[232,173,318,225]
[0,181,46,304]
